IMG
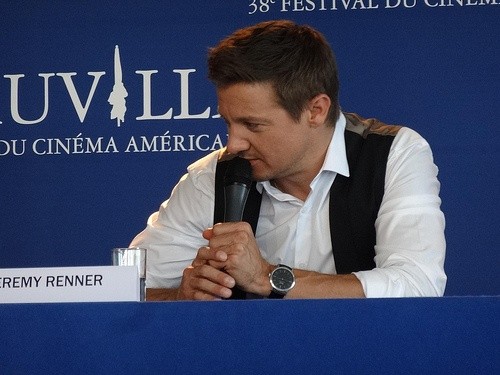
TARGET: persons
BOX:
[120,21,448,301]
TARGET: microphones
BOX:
[220,157,253,272]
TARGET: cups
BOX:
[112,248,147,302]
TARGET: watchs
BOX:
[269,264,296,300]
